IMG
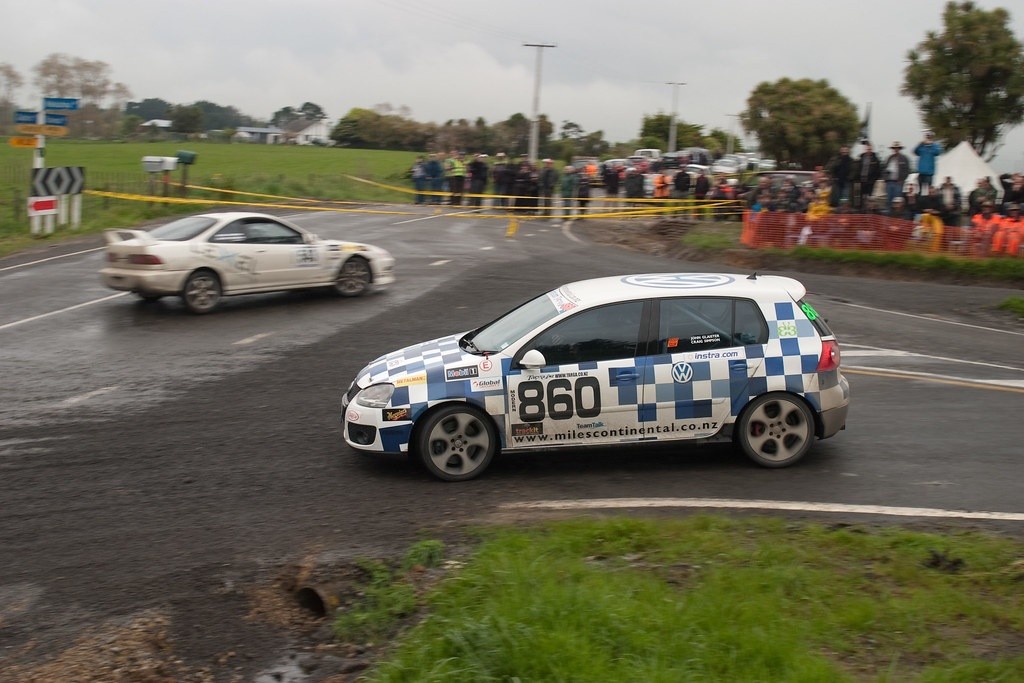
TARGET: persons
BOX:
[695,169,710,221]
[602,166,619,213]
[829,146,853,206]
[738,177,779,247]
[654,172,671,219]
[575,167,591,220]
[772,165,833,247]
[914,132,942,194]
[856,145,881,208]
[826,176,961,253]
[884,141,910,209]
[624,166,644,218]
[492,152,556,216]
[968,169,1024,258]
[410,150,489,213]
[673,164,690,219]
[710,178,738,221]
[559,165,577,220]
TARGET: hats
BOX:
[1006,203,1023,209]
[496,153,505,156]
[892,197,905,203]
[889,142,903,149]
[541,159,554,164]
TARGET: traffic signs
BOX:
[8,96,80,148]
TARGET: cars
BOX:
[342,272,852,483]
[96,212,396,316]
[565,144,777,195]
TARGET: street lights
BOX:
[666,81,686,151]
[523,42,558,161]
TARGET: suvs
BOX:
[703,170,825,219]
